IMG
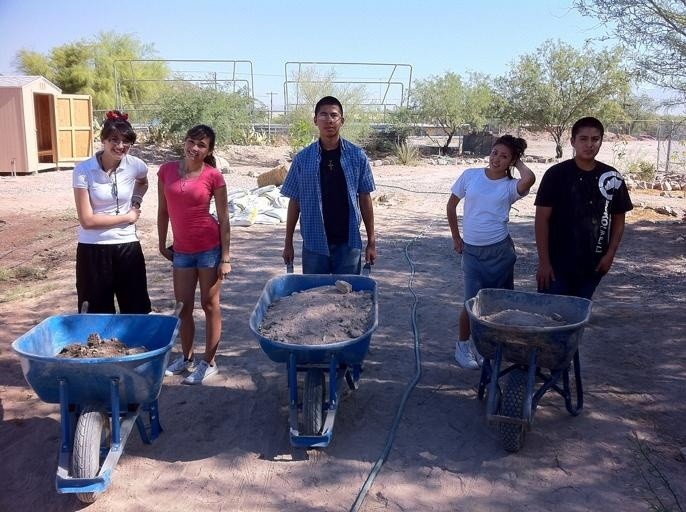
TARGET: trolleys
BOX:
[9,299,185,505]
[463,286,596,454]
[246,259,381,459]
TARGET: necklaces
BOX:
[98,155,106,172]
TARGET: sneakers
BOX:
[455,340,485,369]
[164,355,195,376]
[184,360,218,385]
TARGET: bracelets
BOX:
[219,261,230,263]
[130,195,143,207]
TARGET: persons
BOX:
[156,124,232,385]
[446,133,536,371]
[71,110,152,314]
[279,95,378,275]
[533,117,635,301]
